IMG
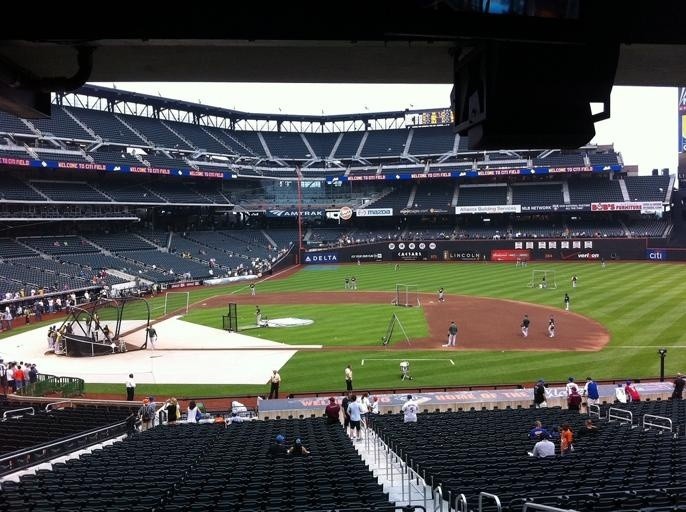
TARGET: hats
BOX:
[538,376,632,392]
[143,395,153,404]
[296,439,302,444]
[328,393,412,405]
[276,435,284,443]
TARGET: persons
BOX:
[0,330,120,396]
[125,372,138,399]
[668,370,686,400]
[267,369,282,400]
[564,376,583,408]
[584,375,600,407]
[533,377,550,409]
[145,322,159,348]
[342,363,355,390]
[518,313,531,338]
[448,320,459,347]
[614,381,627,406]
[547,314,556,338]
[579,417,602,438]
[399,357,414,381]
[625,379,641,403]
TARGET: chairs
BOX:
[230,128,686,275]
[0,396,686,512]
[2,101,268,304]
